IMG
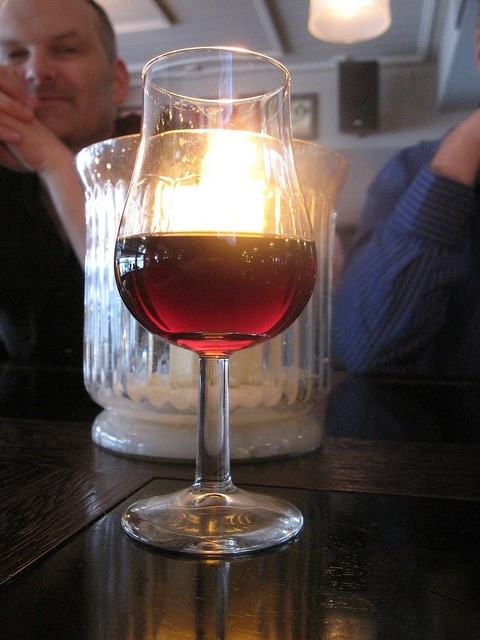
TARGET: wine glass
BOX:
[113,44,316,556]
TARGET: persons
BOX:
[0,0,142,360]
[333,15,480,379]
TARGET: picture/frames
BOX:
[289,93,322,140]
